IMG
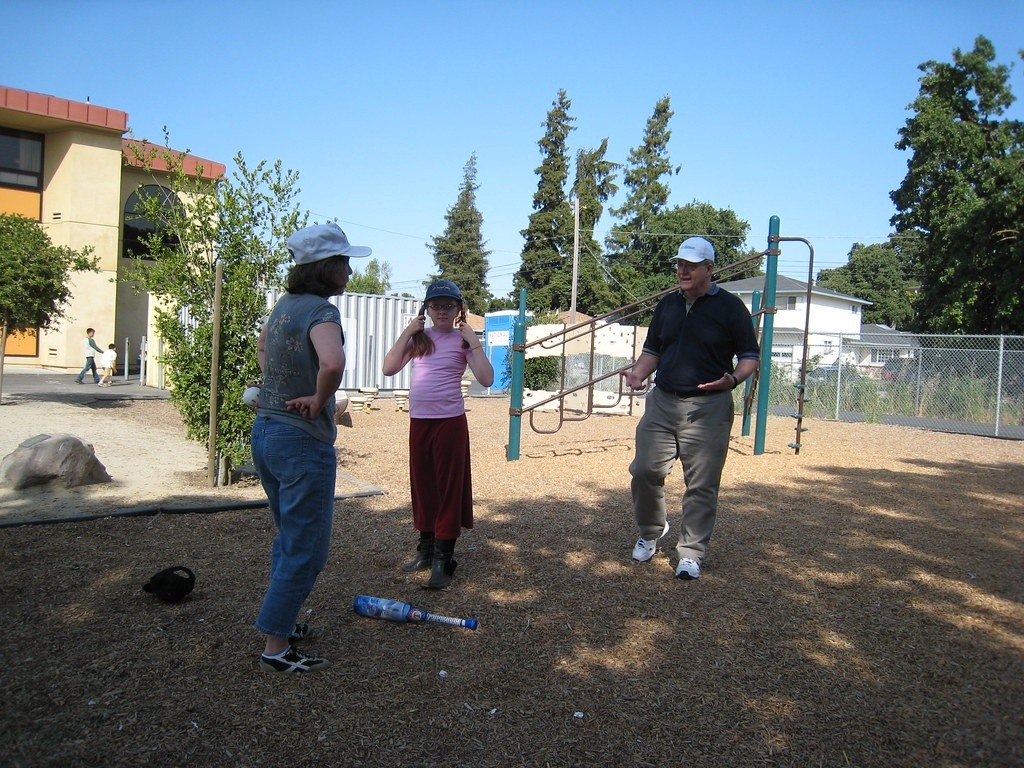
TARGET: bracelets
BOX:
[731,374,739,389]
[472,345,482,351]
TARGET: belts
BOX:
[657,384,719,398]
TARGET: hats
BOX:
[668,236,714,264]
[142,565,195,602]
[423,281,462,306]
[287,224,372,267]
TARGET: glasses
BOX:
[429,303,462,310]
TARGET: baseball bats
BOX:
[352,594,478,631]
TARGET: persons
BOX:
[74,328,104,383]
[621,237,760,579]
[383,280,494,589]
[98,344,117,388]
[247,223,373,676]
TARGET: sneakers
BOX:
[281,623,308,643]
[260,646,330,674]
[675,557,701,580]
[632,521,669,562]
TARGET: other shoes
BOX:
[401,539,433,572]
[429,553,454,588]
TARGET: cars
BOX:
[807,364,857,394]
